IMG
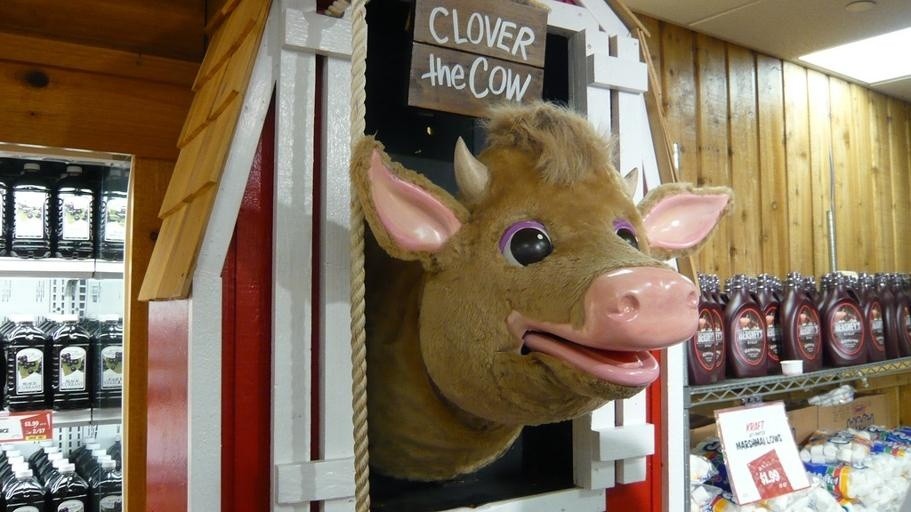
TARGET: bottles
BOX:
[686,264,911,386]
[0,434,122,511]
[0,317,123,411]
[0,160,129,262]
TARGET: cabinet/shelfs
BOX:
[0,157,130,512]
